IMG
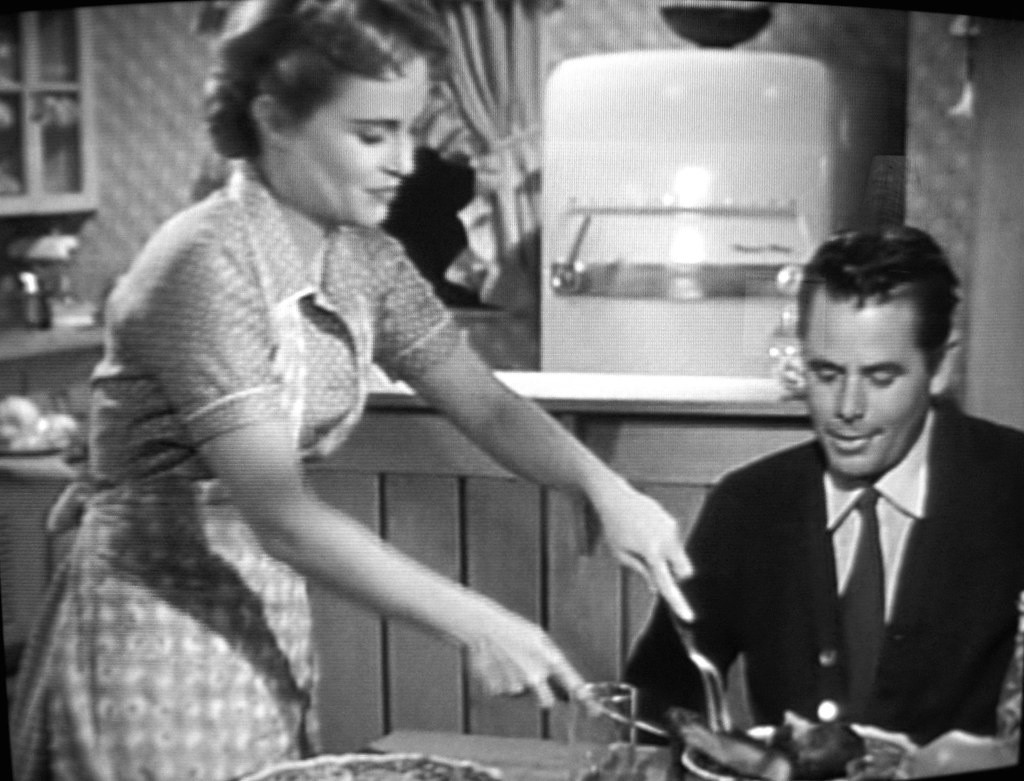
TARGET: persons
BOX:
[617,225,1024,751]
[11,0,696,781]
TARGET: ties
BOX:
[841,487,886,727]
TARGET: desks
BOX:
[364,726,669,781]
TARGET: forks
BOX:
[661,598,734,733]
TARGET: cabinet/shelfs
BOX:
[0,6,100,219]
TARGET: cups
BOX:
[566,682,639,781]
